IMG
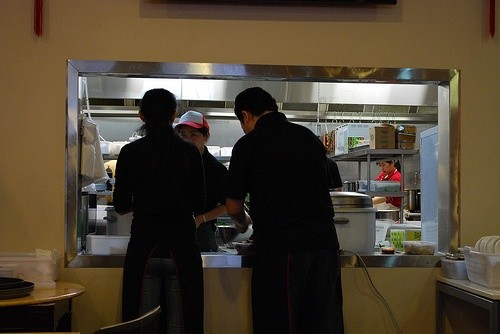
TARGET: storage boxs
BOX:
[335,122,417,149]
[402,240,438,255]
[440,258,468,280]
[0,251,63,282]
[463,246,500,289]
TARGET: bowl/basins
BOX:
[217,226,240,244]
[474,235,500,254]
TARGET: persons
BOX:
[174,111,228,251]
[227,85,346,334]
[112,88,205,334]
[372,160,401,208]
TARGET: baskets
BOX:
[440,256,468,280]
[464,245,500,288]
[0,252,60,282]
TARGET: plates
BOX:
[0,277,34,300]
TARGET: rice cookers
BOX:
[329,192,375,253]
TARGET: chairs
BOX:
[93,305,161,334]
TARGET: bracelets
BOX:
[202,214,206,224]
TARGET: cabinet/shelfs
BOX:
[331,150,420,223]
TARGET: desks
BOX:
[435,275,500,334]
[0,281,87,332]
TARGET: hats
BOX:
[175,110,209,134]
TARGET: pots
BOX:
[376,209,400,221]
[103,208,132,235]
